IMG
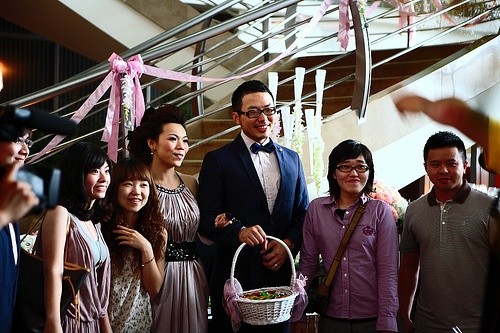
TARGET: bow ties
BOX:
[250,142,276,154]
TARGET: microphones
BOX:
[2,107,78,138]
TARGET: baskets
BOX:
[231,236,297,325]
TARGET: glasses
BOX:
[336,165,369,173]
[15,137,33,148]
[239,107,277,119]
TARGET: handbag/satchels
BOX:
[12,211,91,333]
[311,263,330,313]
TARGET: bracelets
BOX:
[142,257,155,267]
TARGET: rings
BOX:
[274,264,278,267]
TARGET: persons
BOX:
[127,104,231,333]
[298,140,400,333]
[195,79,310,333]
[398,131,500,333]
[394,96,500,175]
[0,120,169,333]
